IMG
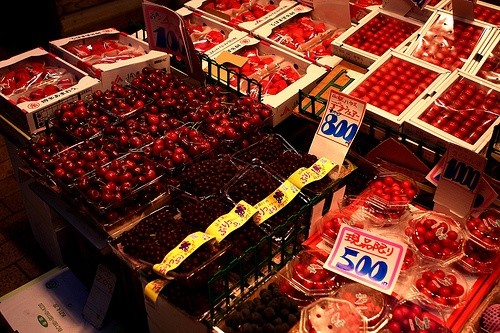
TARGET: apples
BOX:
[0,38,145,105]
[182,0,340,96]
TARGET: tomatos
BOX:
[343,0,500,144]
[280,174,500,333]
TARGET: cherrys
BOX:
[18,67,272,230]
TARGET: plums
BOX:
[226,282,300,333]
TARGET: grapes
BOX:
[121,134,333,312]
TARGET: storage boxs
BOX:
[48,27,171,91]
[165,0,499,153]
[1,45,106,132]
[0,261,109,332]
